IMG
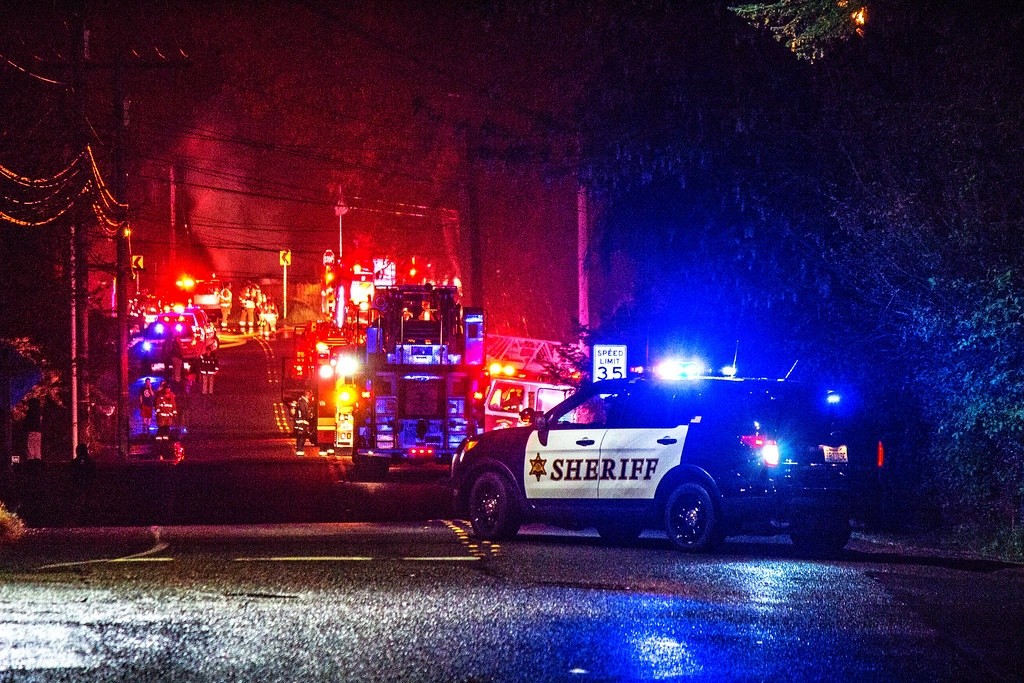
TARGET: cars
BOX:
[125,304,221,458]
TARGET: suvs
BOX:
[448,364,885,555]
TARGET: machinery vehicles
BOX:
[351,276,491,484]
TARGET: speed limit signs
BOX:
[592,343,628,385]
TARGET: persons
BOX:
[139,377,178,435]
[71,443,95,467]
[291,391,315,456]
[170,335,182,383]
[197,346,218,395]
[220,279,279,337]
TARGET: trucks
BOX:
[479,362,578,435]
